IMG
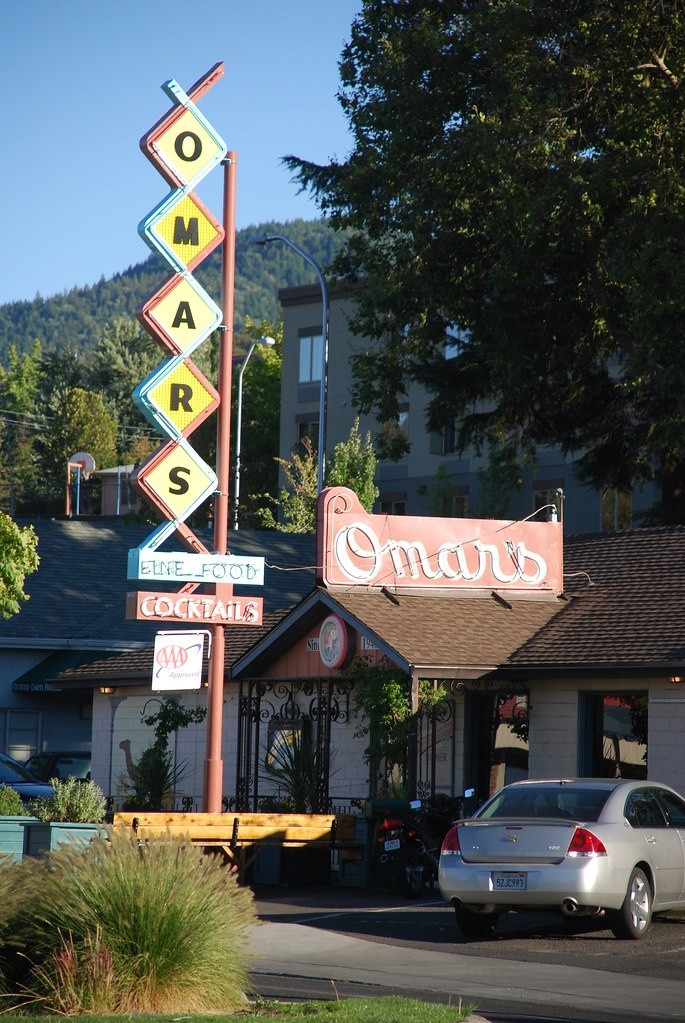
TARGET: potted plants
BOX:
[0,780,116,875]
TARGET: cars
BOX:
[437,779,685,939]
[0,752,56,802]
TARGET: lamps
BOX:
[99,687,116,694]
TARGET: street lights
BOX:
[234,336,276,530]
[247,233,326,493]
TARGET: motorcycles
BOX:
[421,788,476,860]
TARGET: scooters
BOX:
[363,799,439,898]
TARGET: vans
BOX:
[20,751,92,790]
